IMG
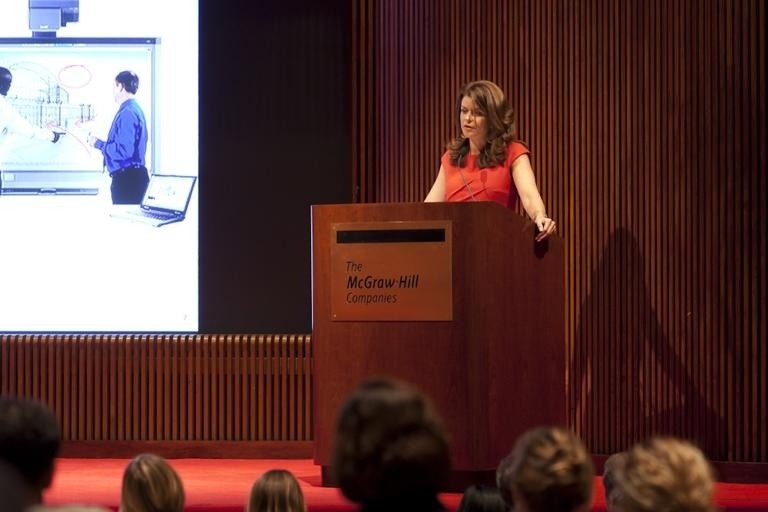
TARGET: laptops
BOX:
[115,173,198,231]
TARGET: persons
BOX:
[248,469,305,512]
[331,378,451,511]
[0,66,67,151]
[457,426,715,511]
[87,69,150,205]
[2,397,59,511]
[422,77,560,244]
[119,454,185,511]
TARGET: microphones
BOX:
[457,147,475,199]
